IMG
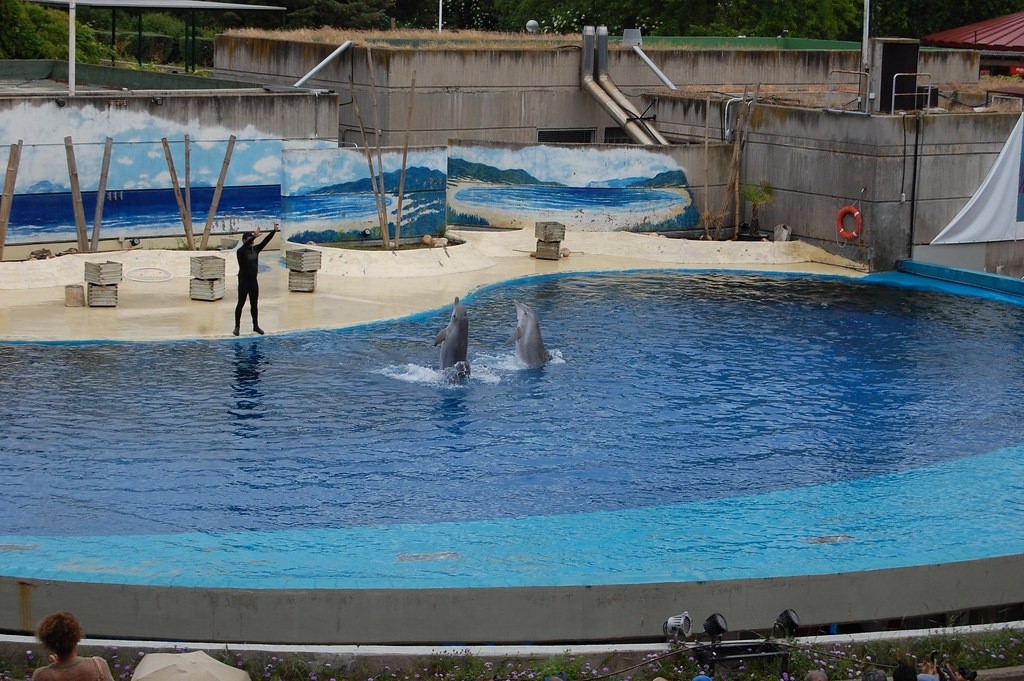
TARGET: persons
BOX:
[233,223,279,336]
[32,612,114,681]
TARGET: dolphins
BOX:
[504,299,549,367]
[433,296,469,372]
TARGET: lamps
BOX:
[772,608,800,644]
[695,613,729,645]
[663,610,693,647]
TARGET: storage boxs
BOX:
[189,256,227,280]
[88,282,118,307]
[285,248,322,271]
[534,221,566,242]
[536,239,561,260]
[85,260,123,285]
[189,277,225,300]
[289,270,317,291]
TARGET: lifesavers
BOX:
[836,205,861,239]
